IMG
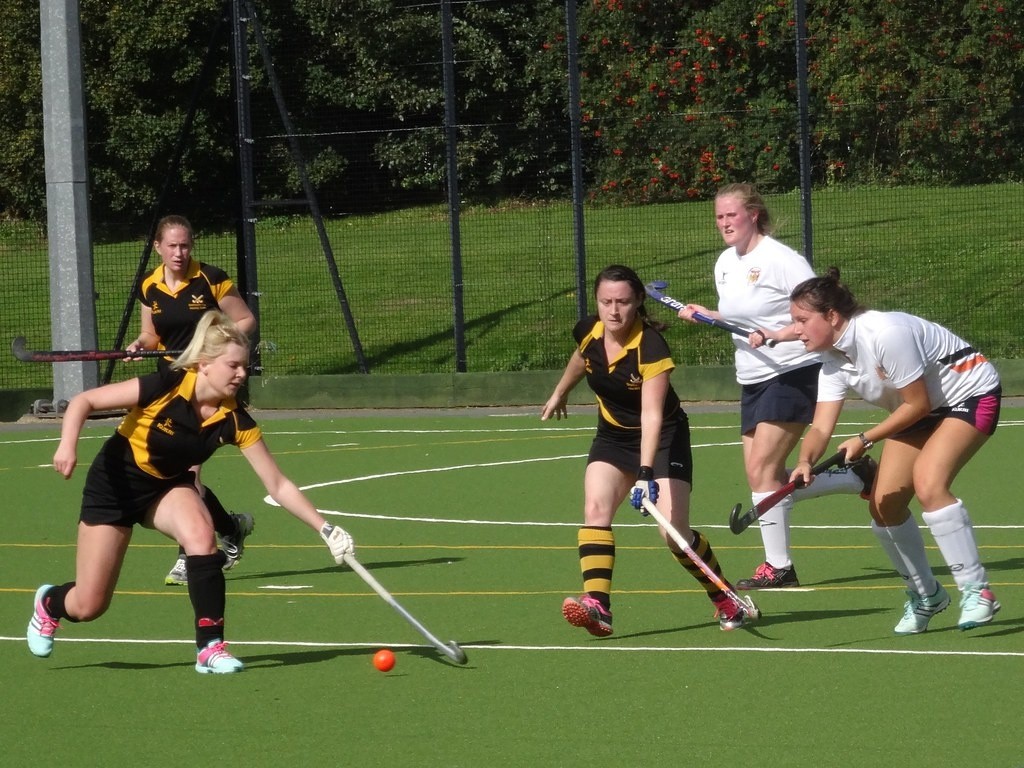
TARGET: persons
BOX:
[789,266,1003,634]
[677,183,878,591]
[539,265,747,637]
[122,216,257,587]
[25,311,356,675]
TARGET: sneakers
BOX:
[195,638,244,674]
[958,580,1001,629]
[895,581,952,635]
[707,579,744,632]
[219,510,255,570]
[851,454,879,501]
[563,593,614,637]
[26,584,63,657]
[164,554,189,587]
[738,559,800,590]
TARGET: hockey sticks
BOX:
[342,552,467,665]
[645,280,777,349]
[630,485,762,621]
[729,446,846,536]
[11,336,187,362]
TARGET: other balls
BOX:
[373,649,397,672]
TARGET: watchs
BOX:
[859,431,873,450]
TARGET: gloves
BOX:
[320,521,356,565]
[630,466,659,518]
